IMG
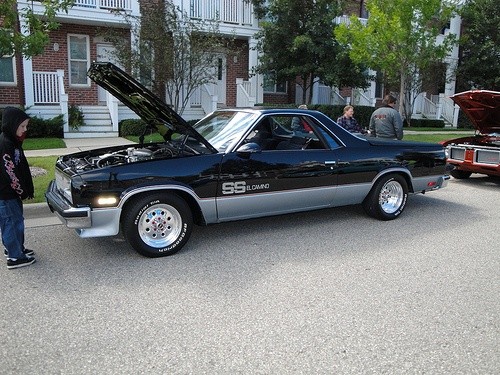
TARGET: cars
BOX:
[438,89,500,184]
[44,61,449,258]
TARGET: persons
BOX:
[337,106,363,134]
[369,95,404,141]
[0,105,36,269]
[291,104,314,133]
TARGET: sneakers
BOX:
[4,248,34,256]
[7,257,36,269]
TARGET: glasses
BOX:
[393,101,396,104]
